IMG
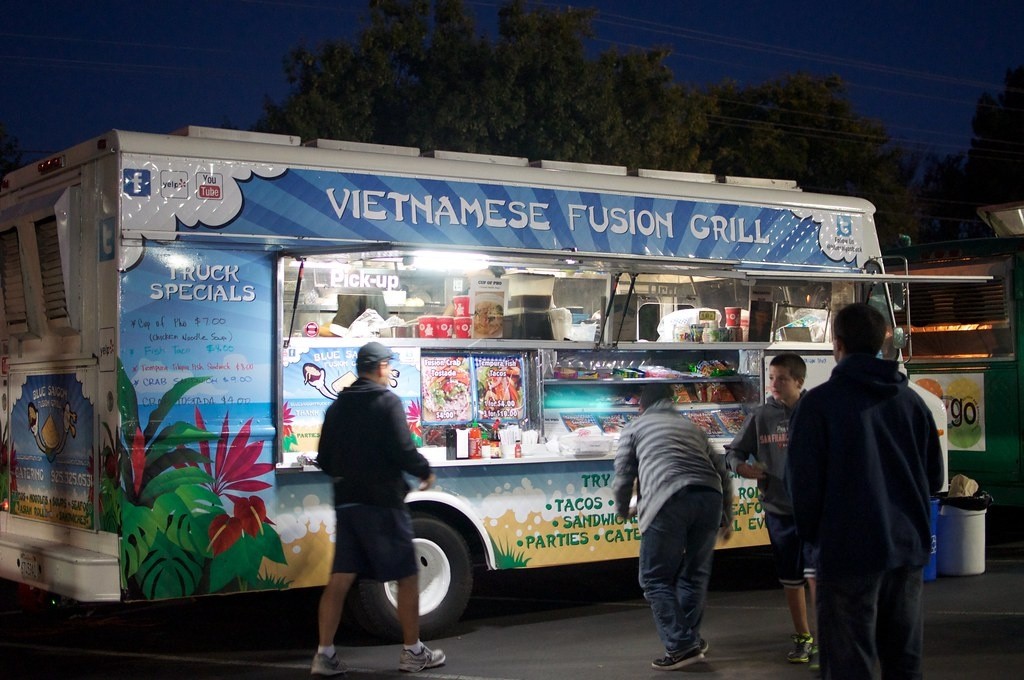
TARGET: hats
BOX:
[356,341,393,366]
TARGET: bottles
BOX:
[468,419,482,459]
[514,440,522,457]
[482,431,491,458]
[490,425,502,458]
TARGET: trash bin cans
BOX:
[921,497,940,581]
[933,490,988,576]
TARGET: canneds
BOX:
[717,327,744,342]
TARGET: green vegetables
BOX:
[431,356,500,411]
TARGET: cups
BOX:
[436,316,453,338]
[453,296,470,317]
[418,316,436,337]
[454,317,472,338]
[725,307,742,327]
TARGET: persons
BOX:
[610,383,733,675]
[310,342,447,677]
[725,353,824,670]
[786,304,945,680]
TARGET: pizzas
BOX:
[473,301,503,333]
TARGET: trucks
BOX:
[1,125,995,643]
[878,200,1024,509]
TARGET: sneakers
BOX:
[808,645,820,670]
[311,652,347,675]
[398,643,445,672]
[788,632,814,663]
[652,647,705,670]
[698,637,708,653]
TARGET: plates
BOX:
[475,293,503,337]
